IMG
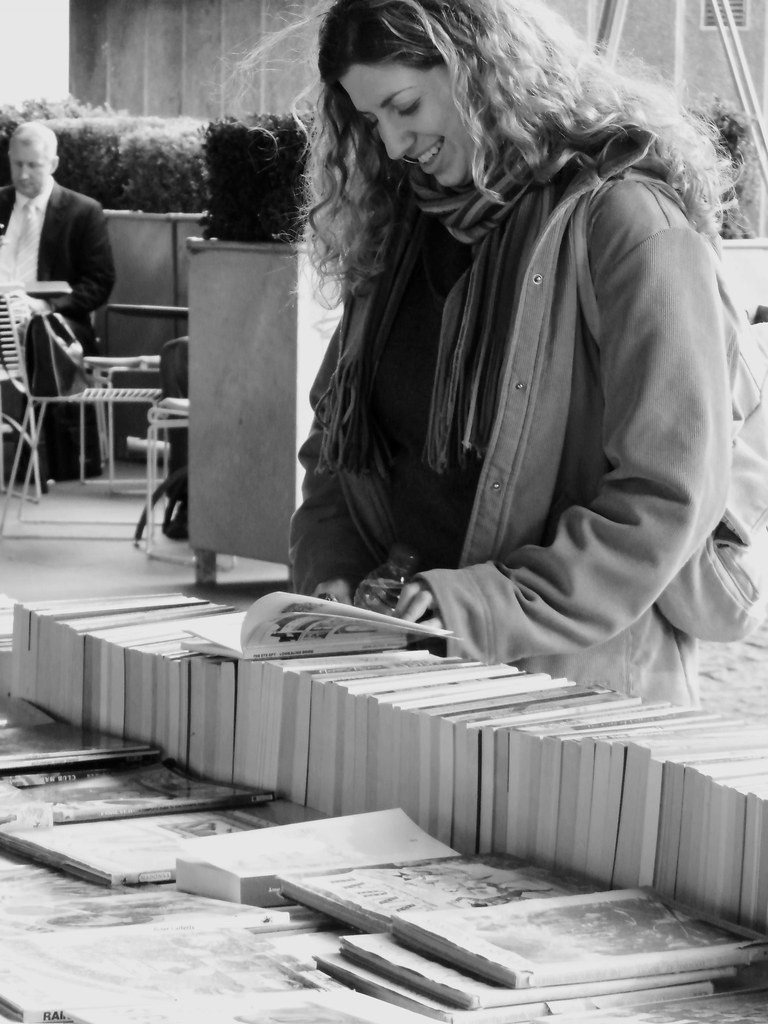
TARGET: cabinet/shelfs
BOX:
[184,235,768,587]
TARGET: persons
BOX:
[288,0,768,709]
[0,123,114,474]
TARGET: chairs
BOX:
[6,290,238,567]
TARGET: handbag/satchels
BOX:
[569,168,768,643]
[23,308,88,396]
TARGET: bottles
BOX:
[354,543,419,619]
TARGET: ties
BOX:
[16,202,40,281]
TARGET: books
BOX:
[1,592,768,1022]
